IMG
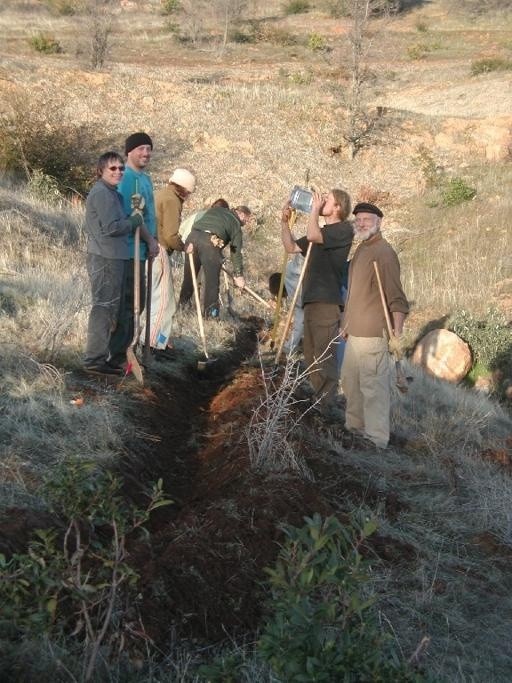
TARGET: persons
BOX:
[269,254,347,384]
[330,203,409,454]
[179,198,229,303]
[282,188,355,411]
[84,151,144,376]
[140,168,195,360]
[106,132,160,373]
[178,206,250,322]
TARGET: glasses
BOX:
[109,166,125,171]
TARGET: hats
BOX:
[169,168,196,193]
[352,202,384,217]
[125,133,153,156]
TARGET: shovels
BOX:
[226,263,236,320]
[188,253,219,372]
[372,259,414,393]
[262,240,312,387]
[127,227,145,385]
[220,264,288,322]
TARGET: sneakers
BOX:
[83,362,123,377]
